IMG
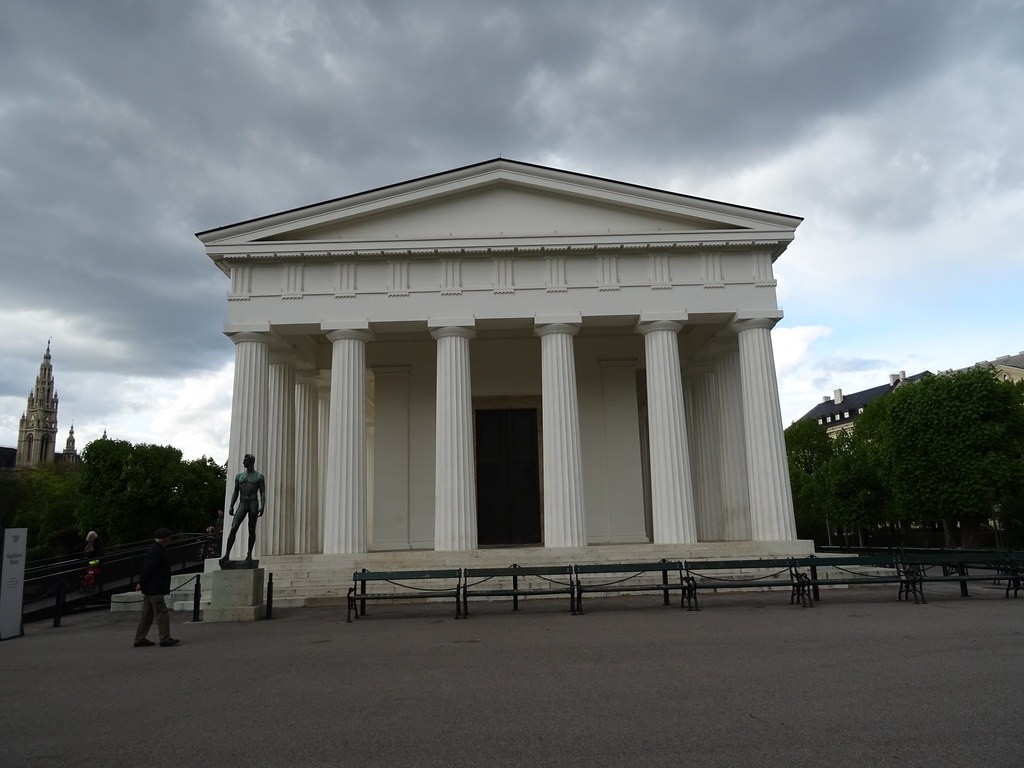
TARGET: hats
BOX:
[153,528,176,539]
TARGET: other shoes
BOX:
[160,637,179,646]
[134,639,155,647]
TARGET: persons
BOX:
[218,454,265,563]
[82,531,105,596]
[197,504,224,562]
[133,528,180,646]
